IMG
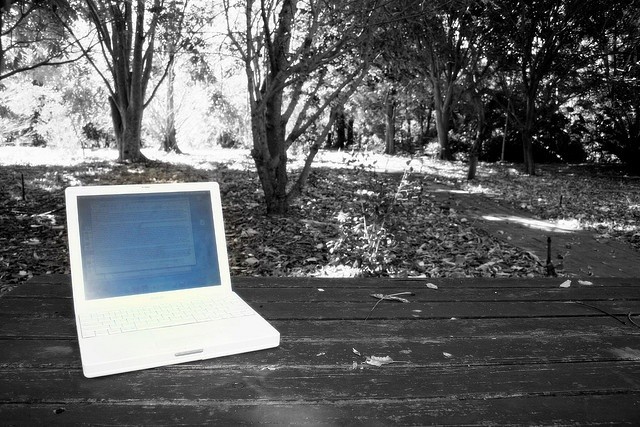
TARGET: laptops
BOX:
[65,182,280,378]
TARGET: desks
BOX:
[0,275,640,426]
